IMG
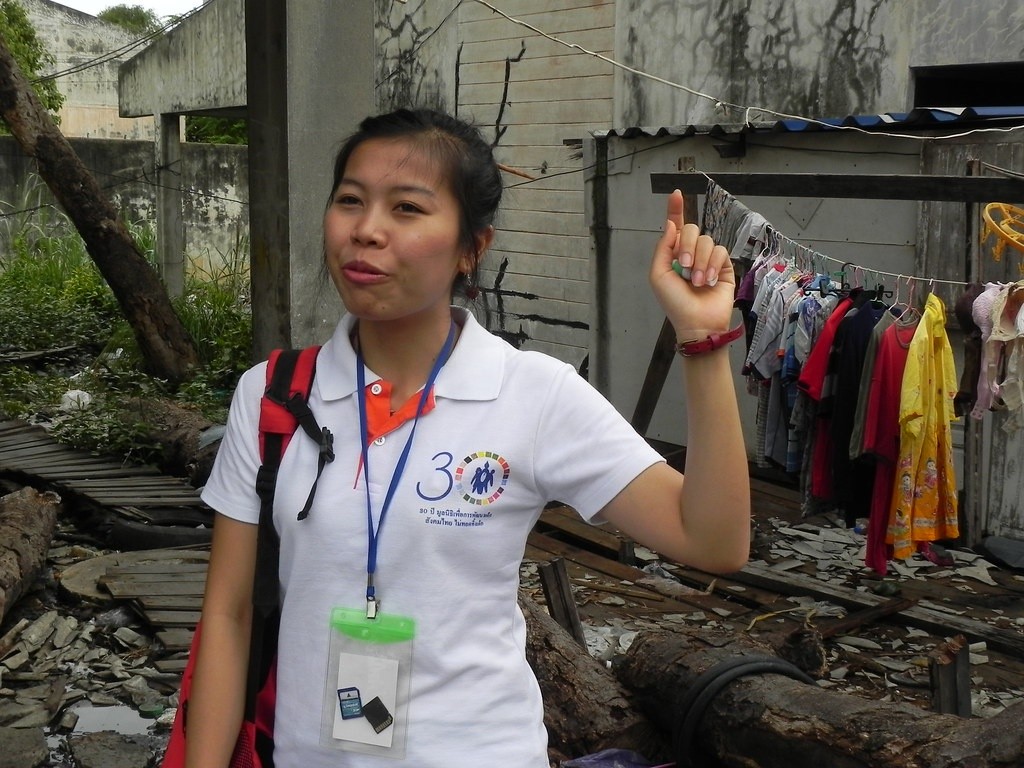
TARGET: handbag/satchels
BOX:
[159,344,321,768]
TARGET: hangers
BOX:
[756,224,935,324]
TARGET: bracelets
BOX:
[675,321,745,357]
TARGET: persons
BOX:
[185,110,752,768]
